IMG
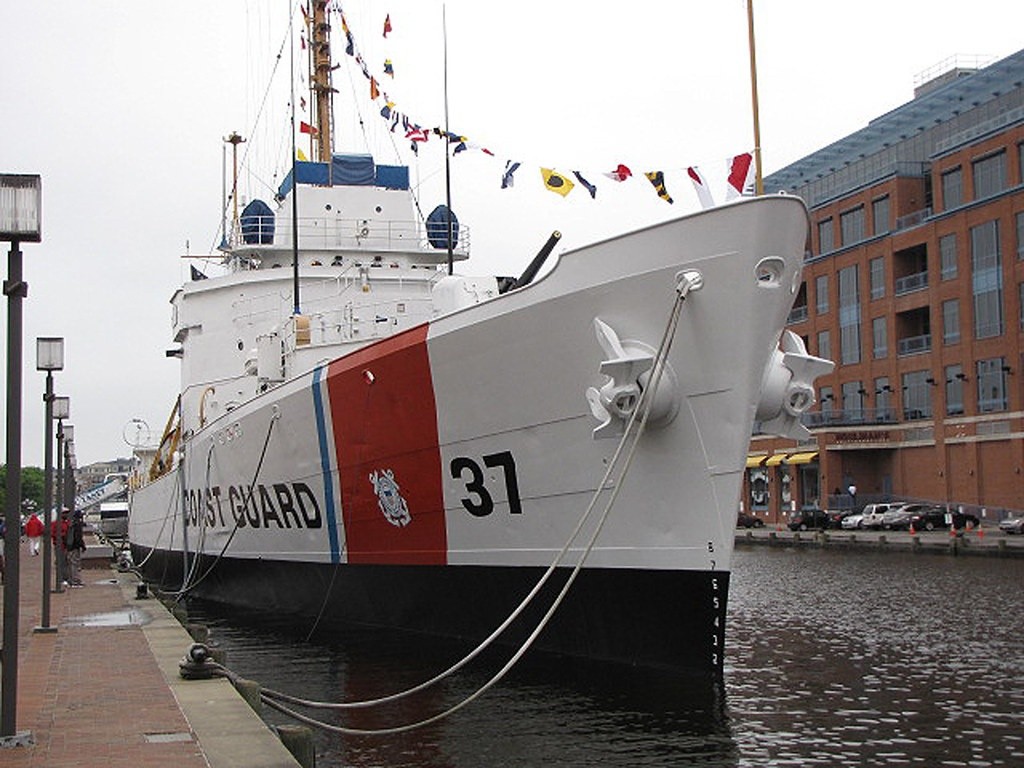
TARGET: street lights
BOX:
[0,173,42,748]
[30,337,65,633]
[52,397,80,593]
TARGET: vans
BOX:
[860,504,901,527]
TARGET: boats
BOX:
[125,0,836,687]
[89,471,127,543]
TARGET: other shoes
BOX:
[68,584,84,587]
[32,554,34,557]
[62,581,68,586]
[35,549,39,555]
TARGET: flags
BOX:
[298,149,305,160]
[540,167,574,197]
[342,16,428,157]
[433,128,520,189]
[573,153,752,199]
[383,14,391,37]
[191,264,208,281]
[300,122,317,133]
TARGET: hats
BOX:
[61,507,71,513]
[73,510,85,517]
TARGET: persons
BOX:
[66,511,86,587]
[26,515,44,556]
[51,510,69,586]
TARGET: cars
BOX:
[842,514,863,529]
[999,514,1024,536]
[736,512,764,527]
[887,502,925,528]
[906,506,979,531]
[794,506,857,529]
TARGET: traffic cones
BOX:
[909,524,915,534]
[977,525,984,538]
[949,524,956,537]
